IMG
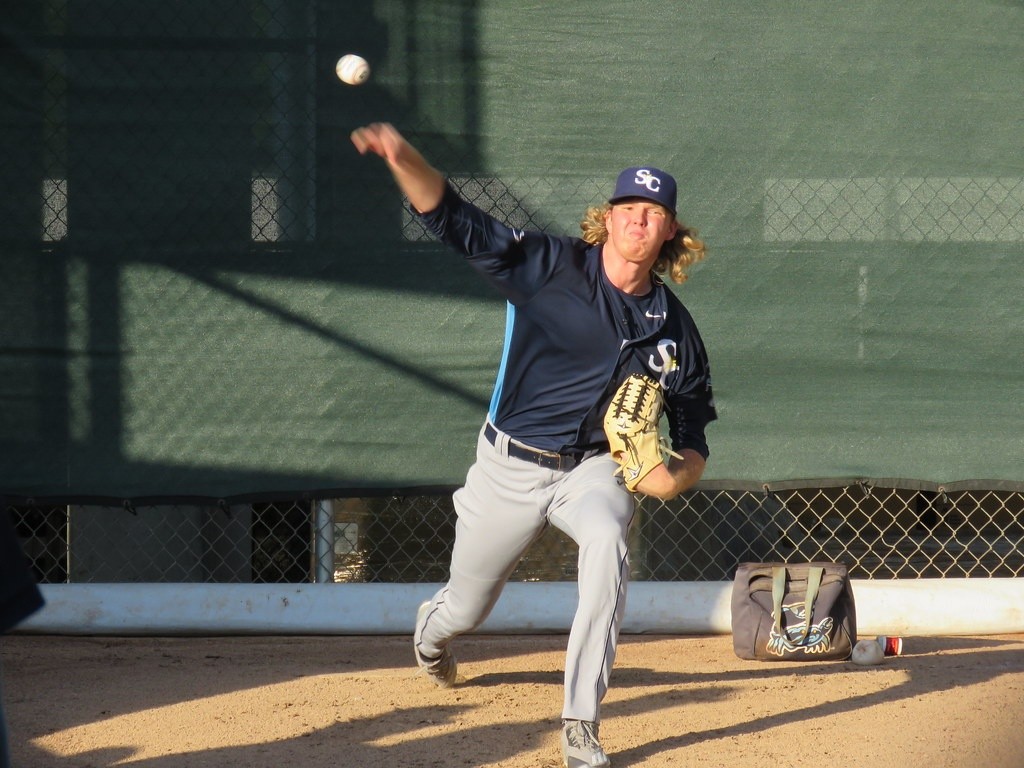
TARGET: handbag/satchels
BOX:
[732,562,857,661]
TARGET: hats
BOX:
[609,167,678,216]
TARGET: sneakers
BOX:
[414,601,458,688]
[561,719,611,768]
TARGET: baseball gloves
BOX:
[601,373,669,493]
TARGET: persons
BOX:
[346,121,715,766]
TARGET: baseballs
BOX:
[336,52,372,89]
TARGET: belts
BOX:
[483,423,582,472]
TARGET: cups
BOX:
[876,636,903,656]
